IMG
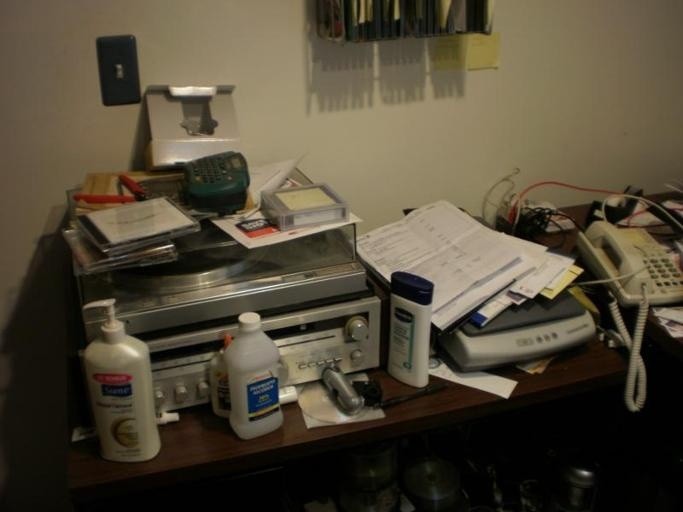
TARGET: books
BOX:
[348,201,584,336]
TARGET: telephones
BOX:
[575,218,683,413]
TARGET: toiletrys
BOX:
[80,296,162,463]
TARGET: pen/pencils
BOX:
[382,384,451,409]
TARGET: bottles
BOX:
[206,333,233,420]
[221,311,285,440]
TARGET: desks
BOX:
[59,199,683,496]
